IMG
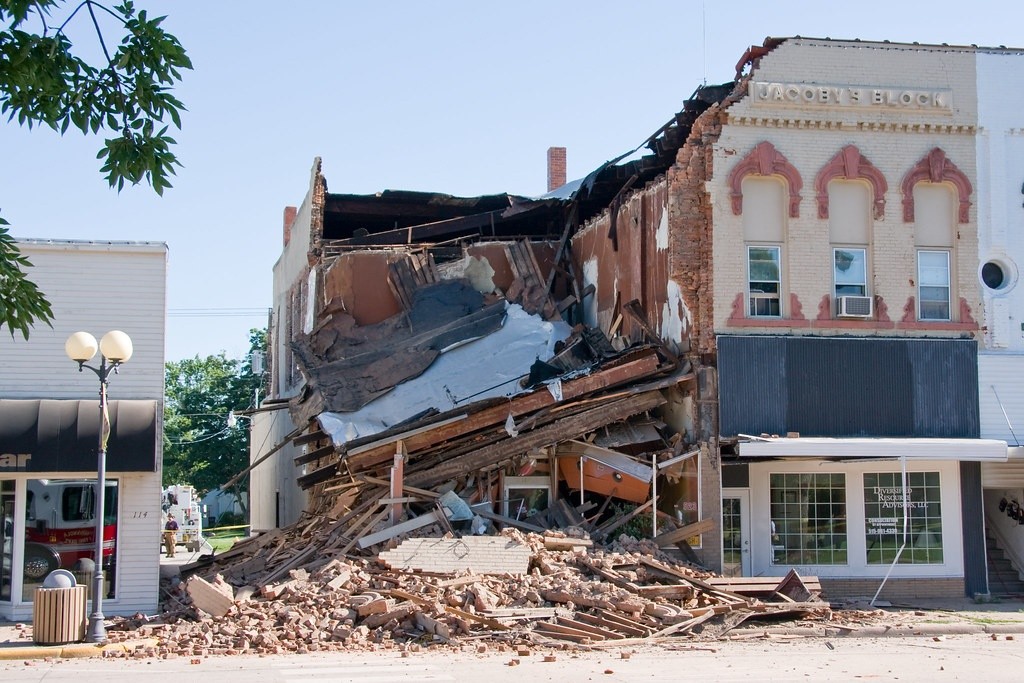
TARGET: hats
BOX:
[167,513,174,518]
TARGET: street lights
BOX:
[64,328,134,646]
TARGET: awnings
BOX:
[0,399,157,471]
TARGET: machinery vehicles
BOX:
[161,483,204,555]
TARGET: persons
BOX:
[162,513,179,557]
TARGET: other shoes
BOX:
[166,555,175,558]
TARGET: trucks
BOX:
[1,480,119,579]
